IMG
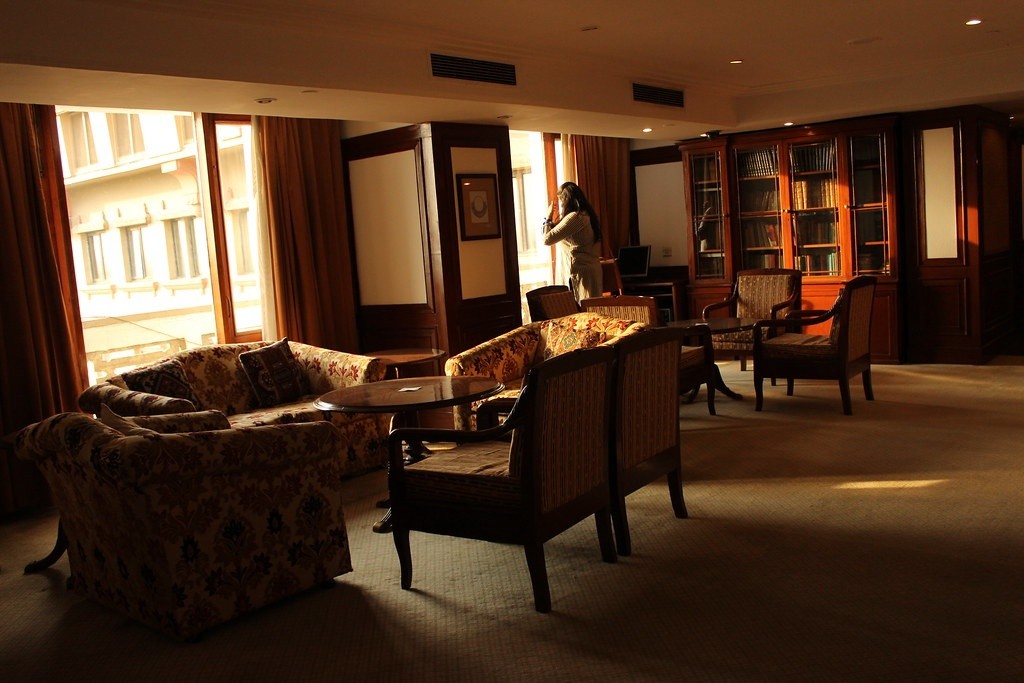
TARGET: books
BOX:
[794,252,841,277]
[740,190,781,211]
[762,254,783,269]
[737,145,779,178]
[744,222,782,248]
[797,222,840,245]
[789,142,836,173]
[791,176,839,216]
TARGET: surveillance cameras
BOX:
[707,131,720,139]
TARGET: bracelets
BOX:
[543,217,553,225]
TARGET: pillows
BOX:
[100,402,160,438]
[121,358,201,408]
[238,337,312,407]
[544,321,608,359]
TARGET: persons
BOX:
[542,182,603,305]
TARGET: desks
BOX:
[311,376,507,533]
[363,347,447,425]
[664,316,760,405]
[623,279,688,322]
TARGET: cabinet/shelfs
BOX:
[674,118,904,364]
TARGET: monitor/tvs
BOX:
[618,245,651,283]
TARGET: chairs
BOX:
[583,295,716,417]
[386,344,617,615]
[599,258,625,296]
[753,276,877,415]
[526,284,578,322]
[603,324,688,556]
[702,268,802,386]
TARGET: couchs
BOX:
[444,310,647,444]
[78,340,389,480]
[7,411,353,643]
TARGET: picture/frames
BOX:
[455,172,502,241]
